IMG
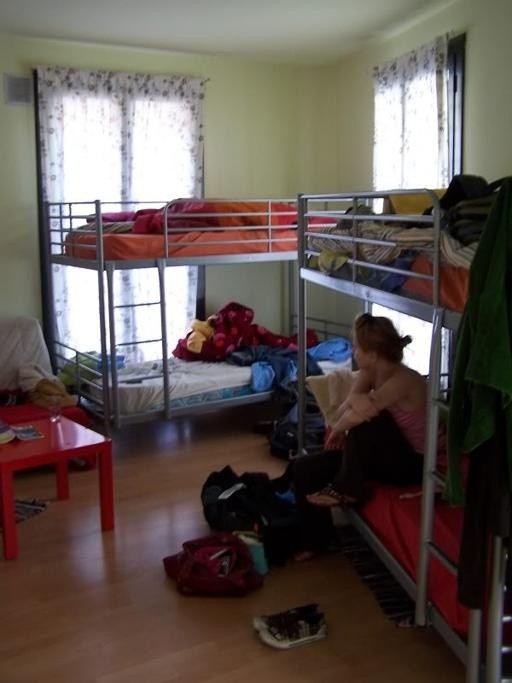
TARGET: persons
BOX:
[305,312,425,507]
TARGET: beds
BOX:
[44,188,361,453]
[297,189,512,683]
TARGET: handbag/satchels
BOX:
[269,413,327,461]
[163,531,266,598]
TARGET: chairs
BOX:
[0,319,98,469]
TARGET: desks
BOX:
[0,414,116,562]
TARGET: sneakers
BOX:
[259,613,328,649]
[253,603,318,632]
